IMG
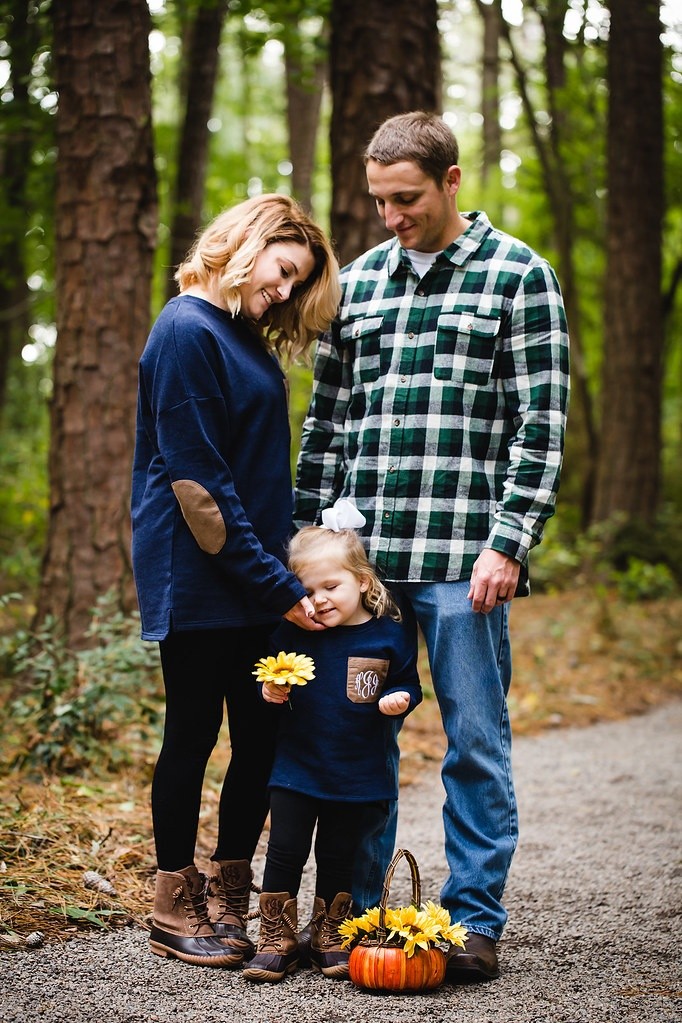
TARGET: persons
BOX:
[293,112,570,980]
[129,194,341,965]
[243,526,424,983]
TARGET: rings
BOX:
[497,594,508,601]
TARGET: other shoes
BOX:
[296,921,313,968]
[446,932,499,979]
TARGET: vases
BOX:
[348,942,446,991]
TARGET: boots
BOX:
[205,857,261,956]
[309,892,353,976]
[243,892,300,982]
[149,865,243,966]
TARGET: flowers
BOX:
[337,901,470,956]
[252,651,315,709]
